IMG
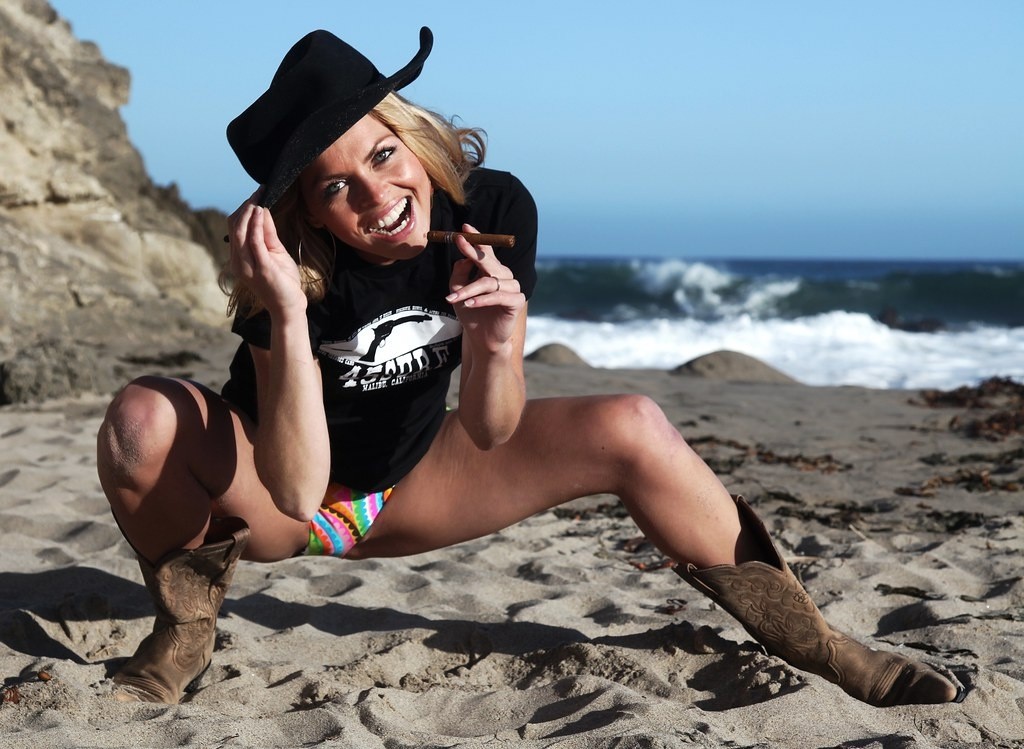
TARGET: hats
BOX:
[224,26,434,243]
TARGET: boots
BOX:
[99,505,251,705]
[672,493,957,708]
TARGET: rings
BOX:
[491,276,499,291]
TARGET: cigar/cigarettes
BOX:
[426,230,515,248]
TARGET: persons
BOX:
[97,28,966,707]
